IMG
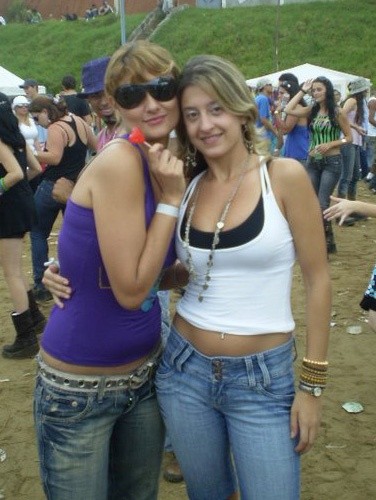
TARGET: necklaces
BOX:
[181,155,253,304]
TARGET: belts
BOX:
[38,363,154,392]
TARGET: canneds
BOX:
[314,145,323,159]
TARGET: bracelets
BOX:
[300,88,307,94]
[156,203,180,216]
[298,358,328,389]
[0,178,9,195]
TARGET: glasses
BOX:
[33,112,42,122]
[283,81,293,91]
[114,78,177,109]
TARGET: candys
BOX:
[128,126,151,147]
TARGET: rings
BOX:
[43,258,60,271]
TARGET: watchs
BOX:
[298,384,322,398]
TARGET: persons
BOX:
[84,0,113,21]
[0,90,47,358]
[250,72,376,253]
[35,39,190,500]
[11,75,98,309]
[0,15,6,26]
[42,52,332,500]
[61,9,80,23]
[25,9,43,23]
[323,195,376,332]
[51,56,127,202]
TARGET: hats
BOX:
[12,96,29,110]
[76,56,110,98]
[257,78,271,91]
[20,79,37,88]
[349,76,372,95]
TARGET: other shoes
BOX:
[30,290,55,307]
[336,216,355,225]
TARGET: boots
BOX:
[3,309,40,357]
[27,290,46,335]
[324,222,335,252]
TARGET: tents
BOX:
[245,61,371,96]
[0,66,47,98]
